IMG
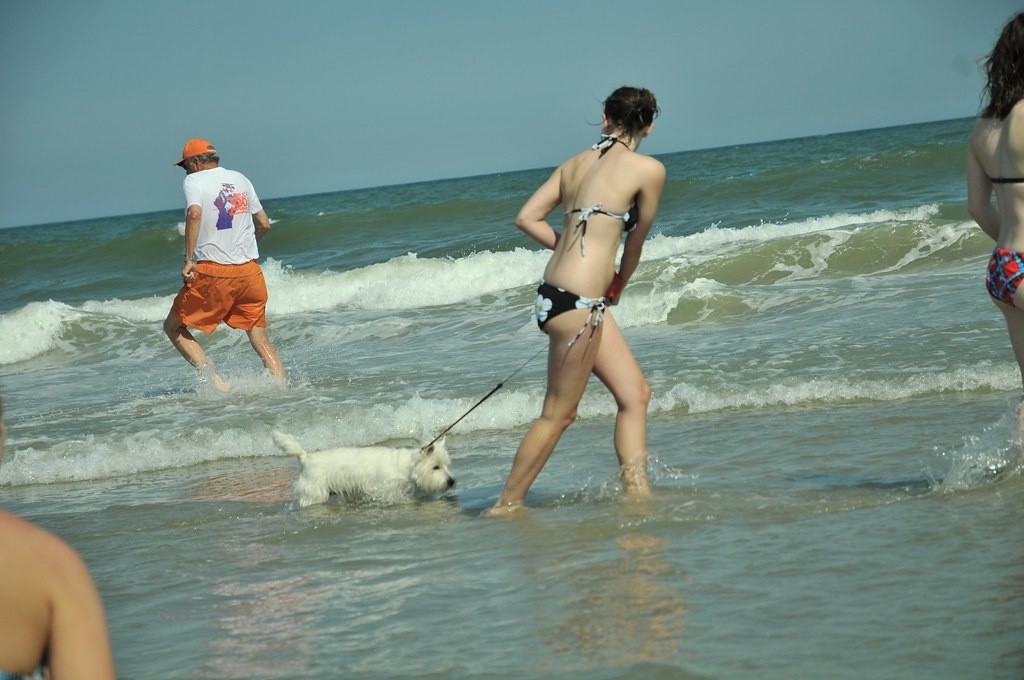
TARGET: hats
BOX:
[173,137,216,166]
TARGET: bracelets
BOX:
[184,255,194,262]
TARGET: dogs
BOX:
[270,430,456,507]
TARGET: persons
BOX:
[163,138,290,392]
[0,394,115,680]
[966,8,1024,431]
[481,85,666,518]
